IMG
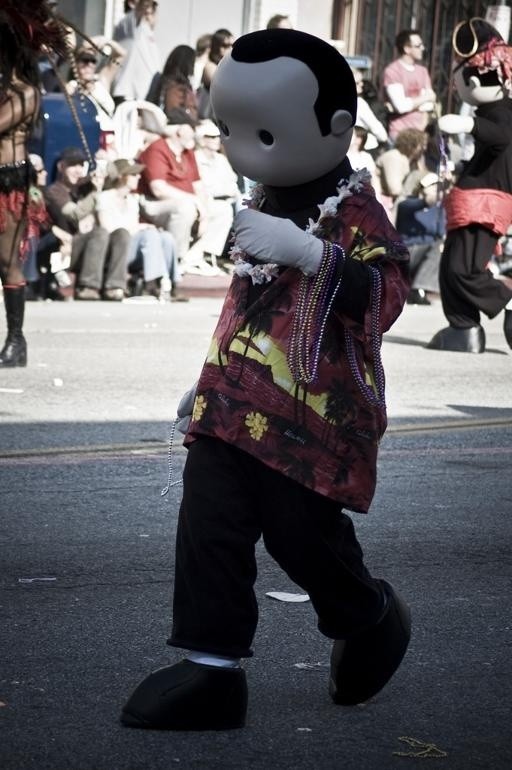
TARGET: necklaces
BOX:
[389,734,447,761]
[160,414,185,498]
[285,234,387,410]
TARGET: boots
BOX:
[0,282,27,369]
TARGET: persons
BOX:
[117,29,412,730]
[0,0,512,373]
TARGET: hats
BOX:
[115,158,145,181]
